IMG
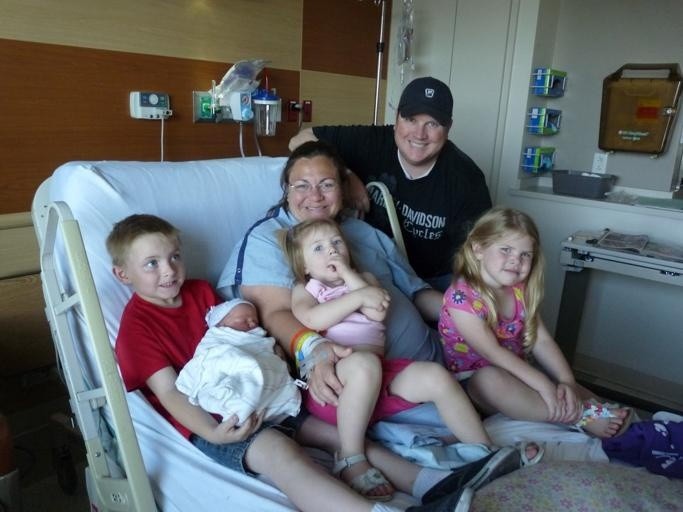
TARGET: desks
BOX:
[554,231,683,368]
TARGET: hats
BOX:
[398,75,455,129]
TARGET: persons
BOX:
[216,142,443,408]
[175,298,301,428]
[285,76,492,296]
[106,214,521,512]
[275,218,545,503]
[438,207,636,441]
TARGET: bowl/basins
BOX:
[550,169,619,199]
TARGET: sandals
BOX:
[570,397,636,440]
[333,449,394,501]
[502,439,545,471]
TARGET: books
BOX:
[596,230,683,263]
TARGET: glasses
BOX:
[285,178,342,194]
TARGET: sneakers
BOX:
[404,487,475,512]
[419,445,520,501]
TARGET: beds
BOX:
[31,157,683,512]
